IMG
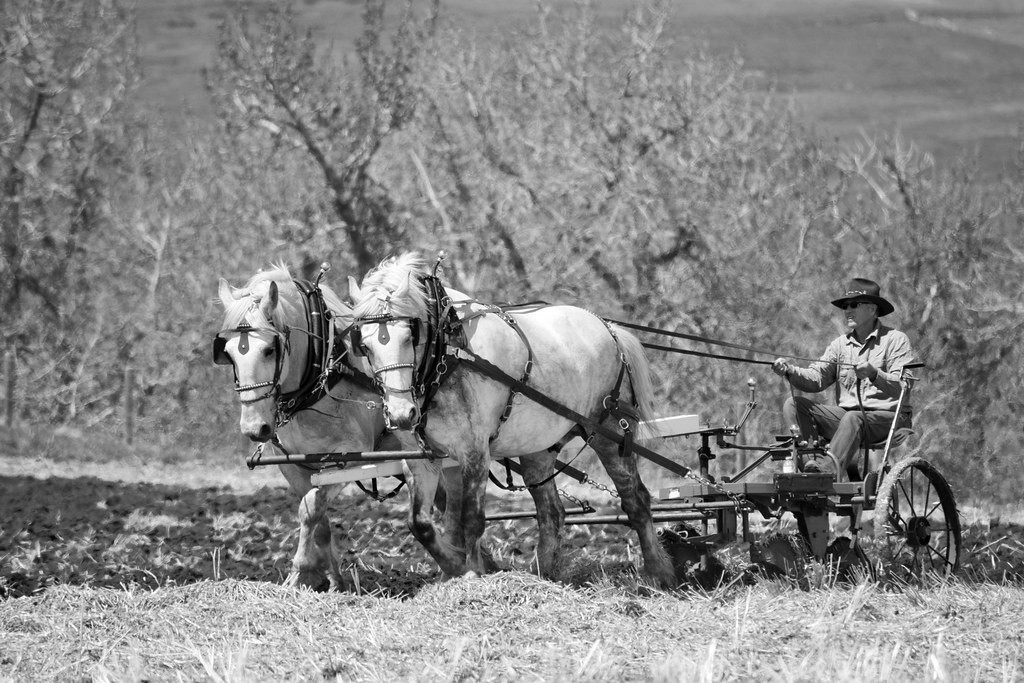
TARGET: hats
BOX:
[830,278,894,317]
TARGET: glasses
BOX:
[841,301,874,311]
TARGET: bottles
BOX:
[783,457,795,473]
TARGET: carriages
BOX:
[214,253,962,590]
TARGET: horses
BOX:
[215,250,680,595]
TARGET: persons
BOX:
[771,278,926,484]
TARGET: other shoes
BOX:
[804,460,843,482]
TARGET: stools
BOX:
[871,427,916,449]
